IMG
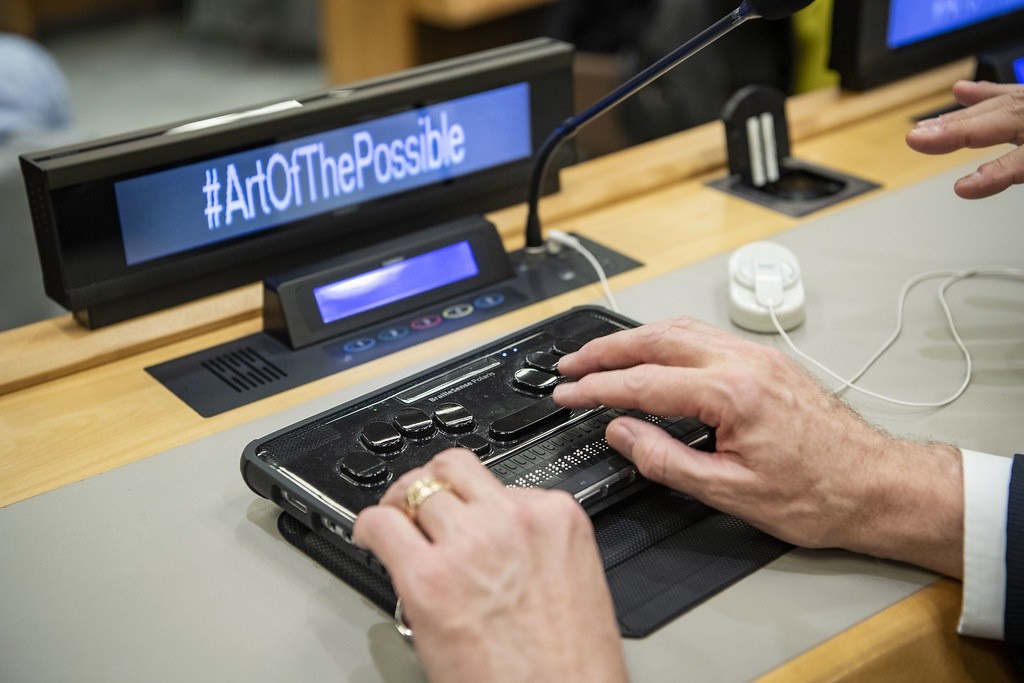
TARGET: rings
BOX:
[407,478,445,508]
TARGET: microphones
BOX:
[525,0,815,253]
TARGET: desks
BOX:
[0,60,1024,683]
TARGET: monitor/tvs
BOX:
[826,0,1024,93]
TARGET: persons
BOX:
[352,316,1024,683]
[421,0,802,141]
